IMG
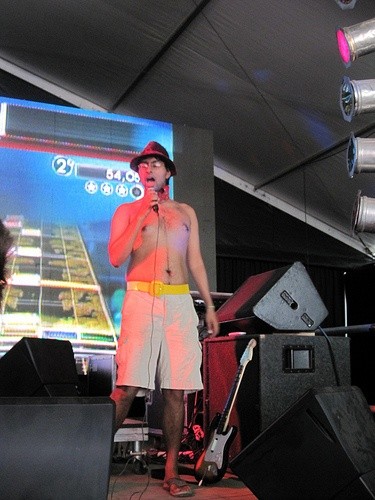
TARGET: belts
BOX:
[126,280,190,299]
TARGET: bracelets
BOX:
[206,305,215,309]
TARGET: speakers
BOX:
[0,337,116,500]
[202,261,373,500]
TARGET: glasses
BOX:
[138,162,161,168]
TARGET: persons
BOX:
[0,220,13,301]
[107,141,221,496]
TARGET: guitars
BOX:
[192,338,257,487]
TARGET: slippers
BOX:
[163,477,192,497]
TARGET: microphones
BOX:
[147,187,159,212]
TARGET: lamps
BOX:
[351,190,375,237]
[345,131,375,178]
[335,18,375,69]
[339,75,375,124]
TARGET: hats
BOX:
[130,141,177,177]
